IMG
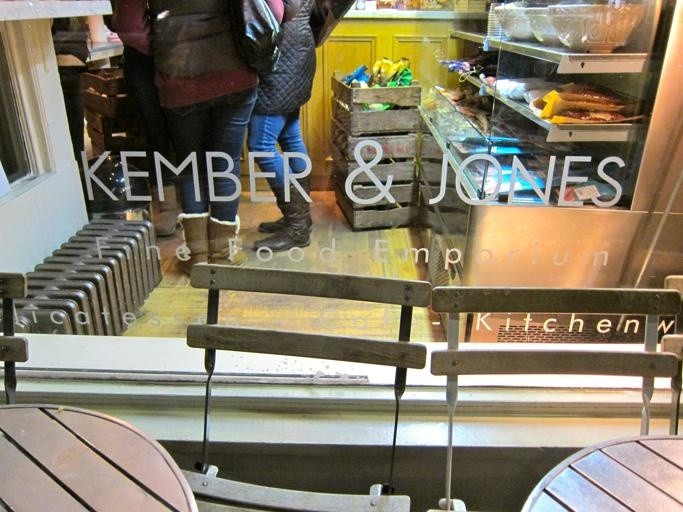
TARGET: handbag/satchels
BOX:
[308,0,357,49]
[227,0,284,76]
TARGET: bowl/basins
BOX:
[493,3,645,53]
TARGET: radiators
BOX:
[1,219,162,337]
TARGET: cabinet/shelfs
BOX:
[240,18,487,192]
[402,3,683,341]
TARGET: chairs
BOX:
[180,259,682,509]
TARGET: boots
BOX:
[255,172,312,234]
[207,214,248,268]
[171,212,209,274]
[253,185,311,252]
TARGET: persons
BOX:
[99,1,186,239]
[99,2,286,277]
[52,17,93,159]
[249,0,314,254]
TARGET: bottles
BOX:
[487,3,506,38]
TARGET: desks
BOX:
[0,407,196,510]
